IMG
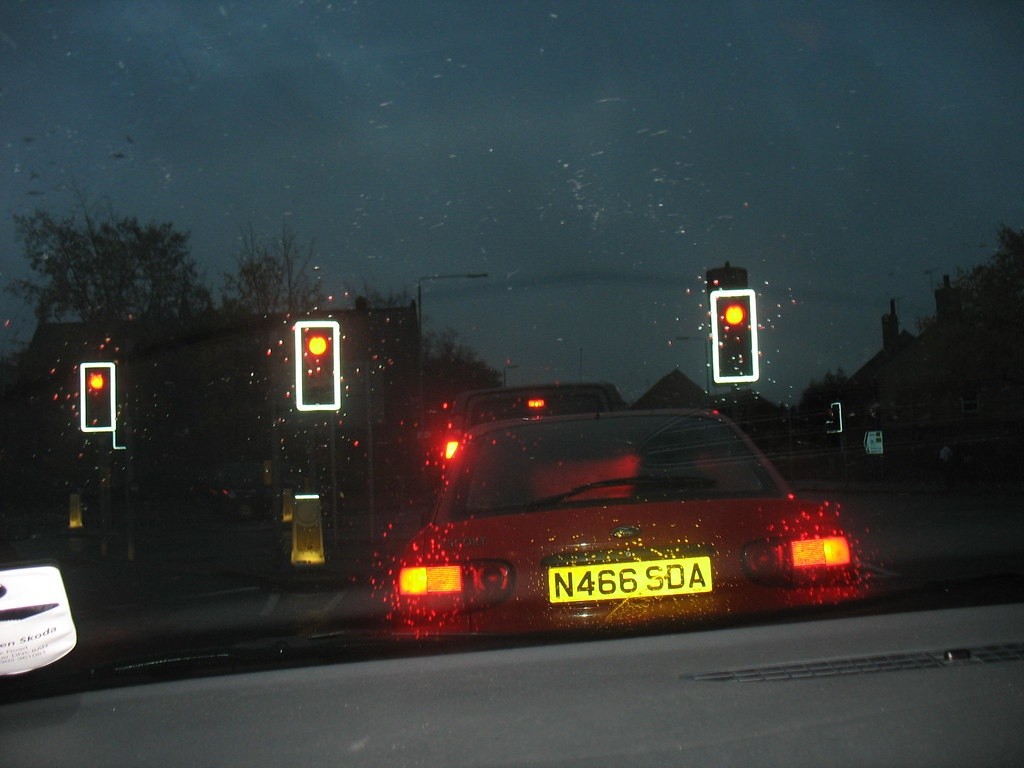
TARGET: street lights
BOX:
[415,272,490,449]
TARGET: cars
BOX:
[389,404,882,647]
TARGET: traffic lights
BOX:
[295,320,341,412]
[711,287,760,383]
[80,362,118,433]
